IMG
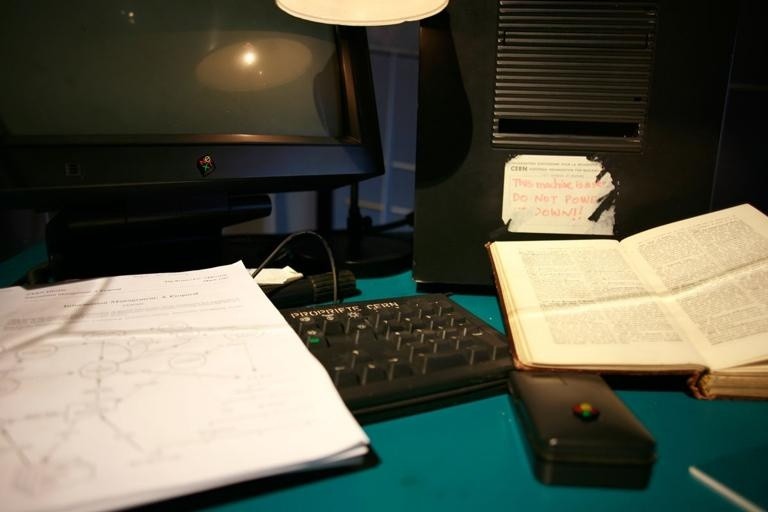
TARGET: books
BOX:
[487,203,767,399]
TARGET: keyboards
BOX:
[278,293,514,426]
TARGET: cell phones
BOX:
[507,367,655,489]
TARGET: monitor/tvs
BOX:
[0,0,384,288]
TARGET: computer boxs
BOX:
[414,0,659,291]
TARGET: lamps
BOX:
[195,1,314,94]
